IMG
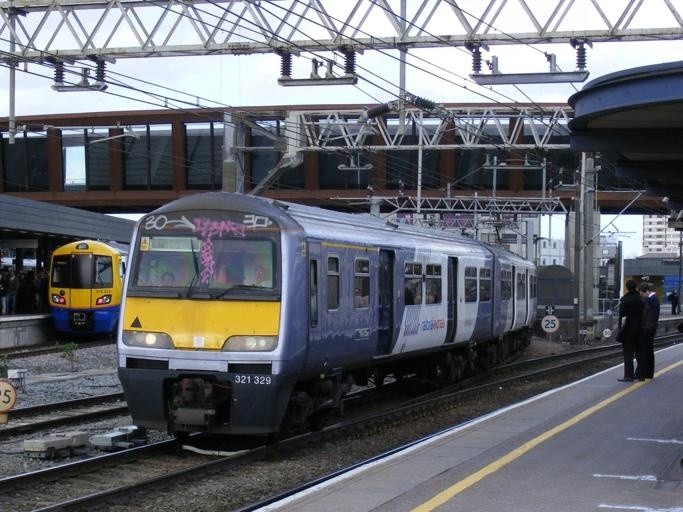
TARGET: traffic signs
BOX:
[539,314,559,333]
[0,379,17,415]
[601,330,611,338]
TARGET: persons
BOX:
[160,272,175,288]
[246,266,272,288]
[616,279,645,382]
[410,287,438,305]
[354,287,370,308]
[635,283,660,379]
[0,261,50,315]
[664,288,679,315]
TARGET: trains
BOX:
[113,191,539,448]
[46,238,130,339]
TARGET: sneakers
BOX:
[617,373,654,382]
